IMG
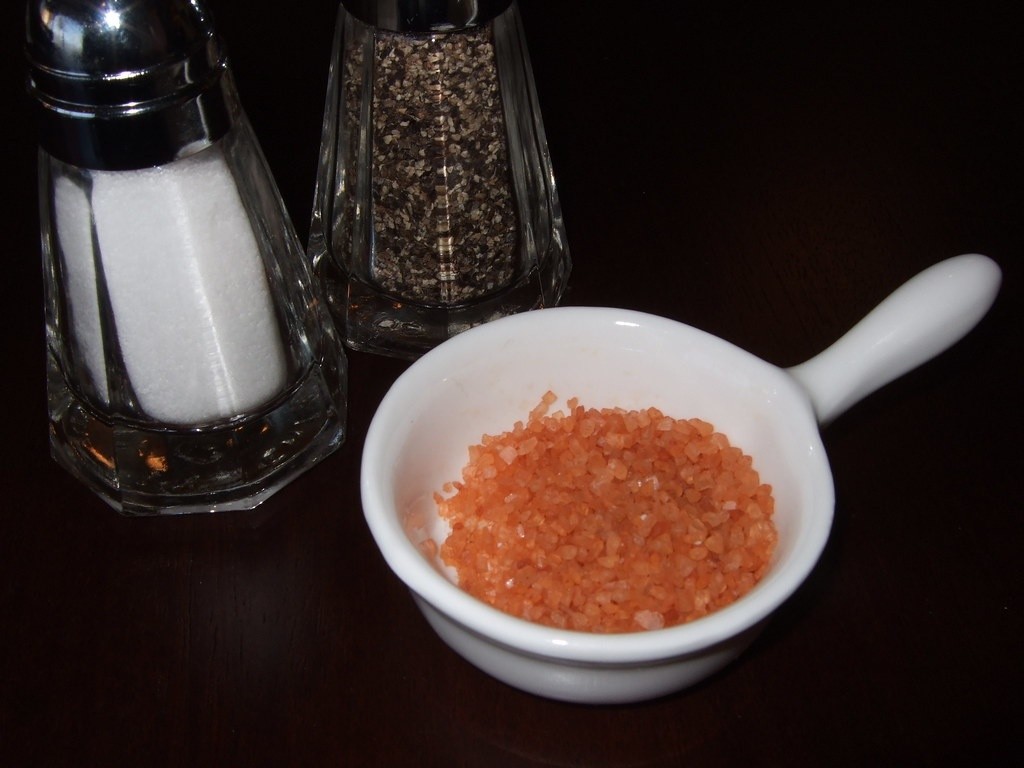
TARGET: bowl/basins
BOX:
[358,256,1003,704]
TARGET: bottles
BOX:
[300,1,575,375]
[13,0,347,518]
